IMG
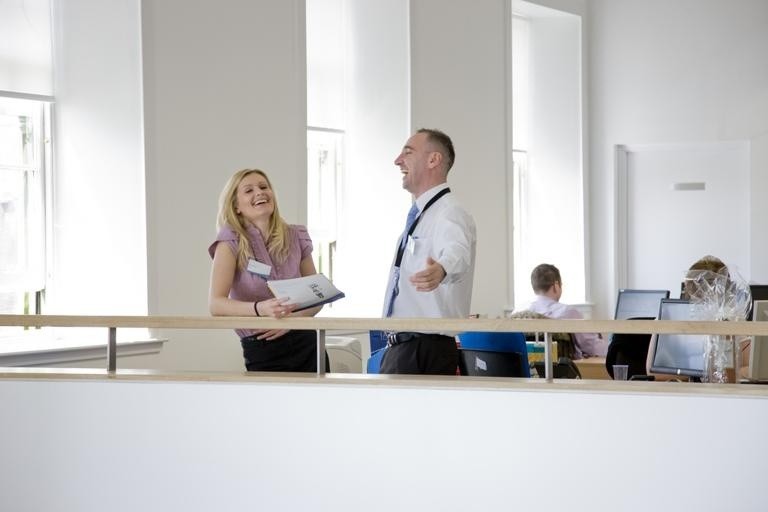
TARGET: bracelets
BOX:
[254,302,259,316]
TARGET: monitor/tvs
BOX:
[748,284,767,319]
[649,297,722,382]
[611,288,669,335]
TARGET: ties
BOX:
[381,204,418,317]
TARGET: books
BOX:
[266,272,345,313]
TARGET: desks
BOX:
[547,356,694,381]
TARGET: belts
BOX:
[388,333,425,344]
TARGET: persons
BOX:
[379,128,477,374]
[683,253,756,321]
[208,168,330,373]
[512,263,606,357]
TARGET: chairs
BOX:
[507,311,577,376]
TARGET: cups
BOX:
[613,365,628,380]
